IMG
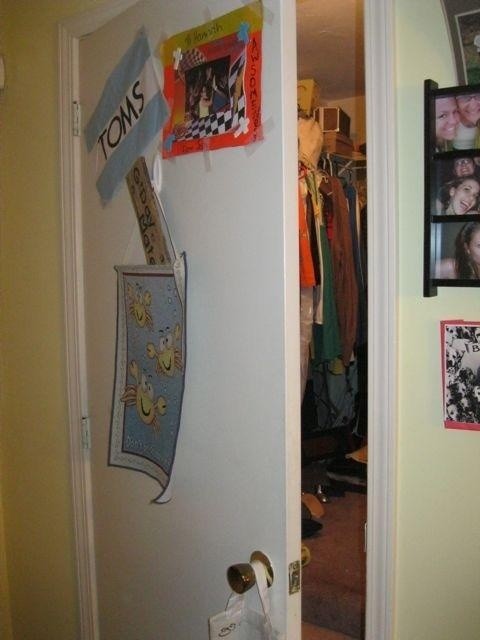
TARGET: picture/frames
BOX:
[423,77,480,298]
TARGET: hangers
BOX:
[298,143,356,191]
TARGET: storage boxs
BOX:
[297,78,322,116]
[312,105,351,138]
[323,132,354,157]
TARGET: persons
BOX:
[436,222,479,282]
[447,92,480,150]
[441,176,480,218]
[186,61,228,119]
[449,152,480,176]
[433,95,462,152]
